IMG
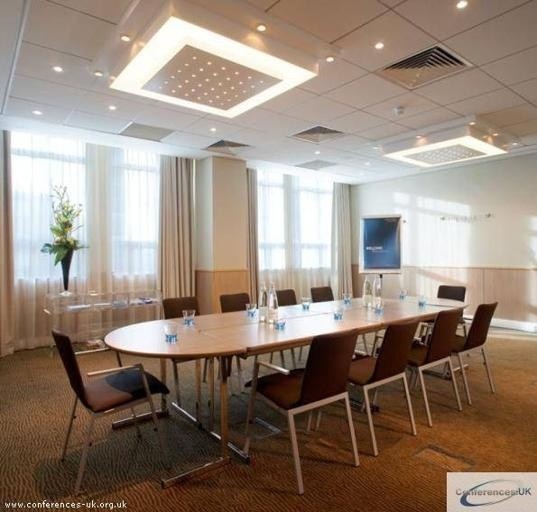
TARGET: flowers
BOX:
[40,184,90,265]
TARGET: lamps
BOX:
[372,115,518,169]
[108,1,319,119]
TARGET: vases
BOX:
[58,249,76,296]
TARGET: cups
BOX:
[332,301,344,321]
[182,310,195,328]
[246,304,257,320]
[272,313,288,330]
[373,297,385,317]
[418,295,428,311]
[301,298,312,310]
[164,322,177,343]
[343,293,353,306]
[399,290,408,303]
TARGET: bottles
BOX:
[361,275,373,308]
[372,274,384,310]
[267,282,279,324]
[258,282,268,323]
